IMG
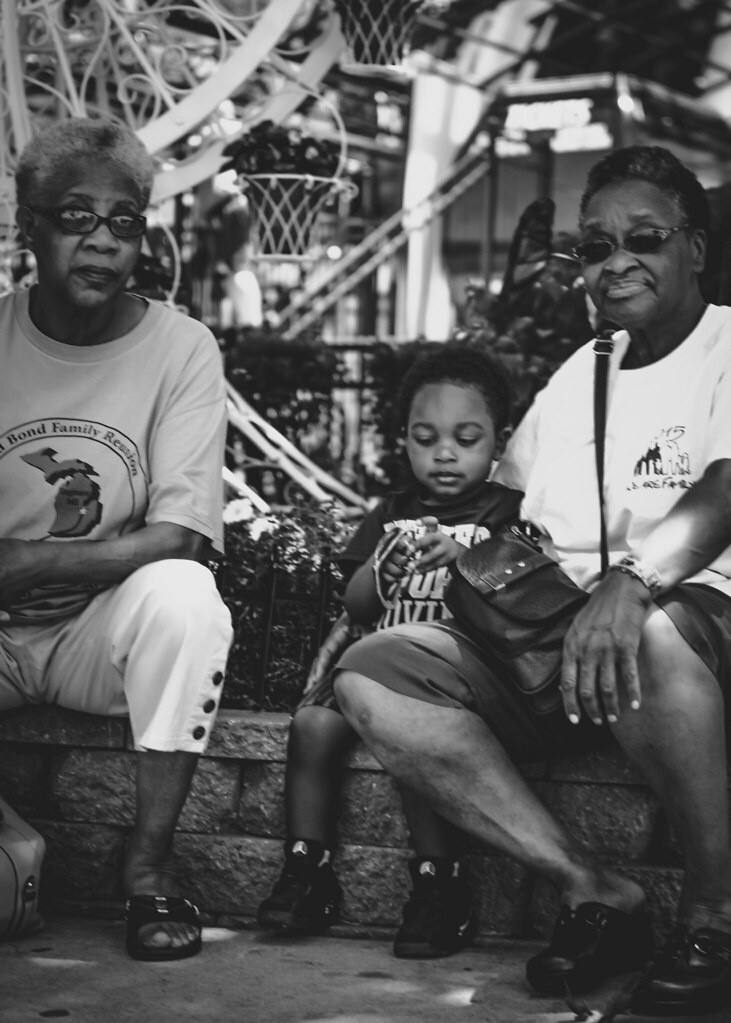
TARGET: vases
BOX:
[335,0,417,69]
[238,175,322,258]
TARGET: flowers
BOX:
[215,118,368,179]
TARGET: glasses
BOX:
[572,225,688,263]
[27,203,147,239]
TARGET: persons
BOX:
[302,147,731,1022]
[250,346,523,959]
[1,115,237,959]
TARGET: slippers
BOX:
[124,893,204,959]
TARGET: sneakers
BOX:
[257,837,342,931]
[394,856,478,958]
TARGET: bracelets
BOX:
[608,553,665,603]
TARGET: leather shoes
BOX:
[525,895,652,993]
[629,918,731,1015]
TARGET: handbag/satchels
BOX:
[445,528,599,718]
[0,791,46,944]
